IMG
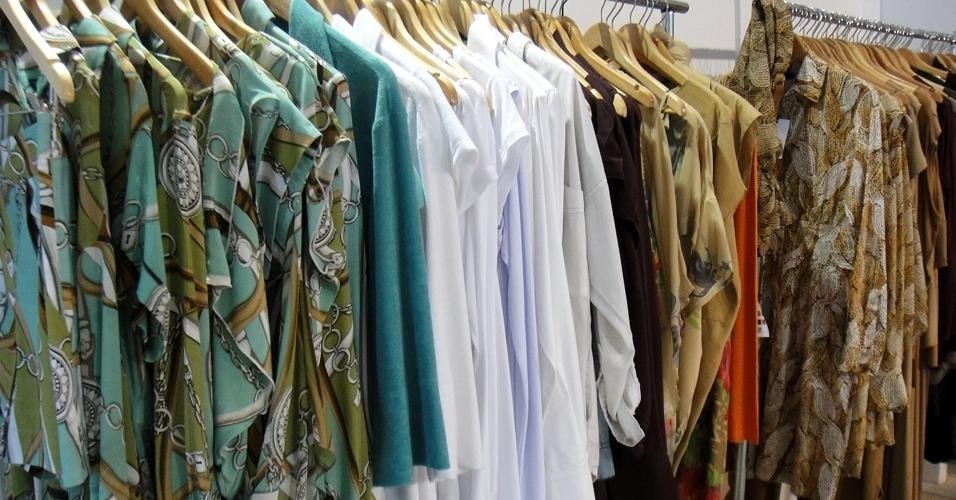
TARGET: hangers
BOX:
[785,3,956,113]
[1,0,688,116]
